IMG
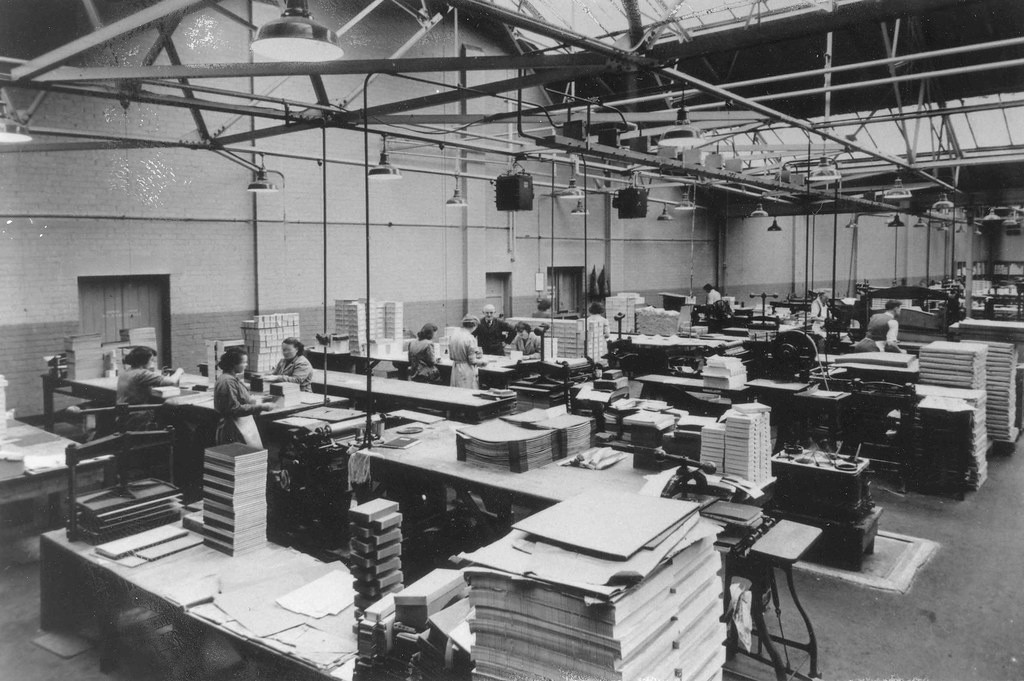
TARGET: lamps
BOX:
[887,210,905,229]
[0,88,34,142]
[750,197,769,218]
[913,216,928,229]
[974,227,982,235]
[368,134,403,180]
[846,219,859,228]
[956,224,965,233]
[656,202,675,221]
[674,185,696,211]
[937,222,949,232]
[983,208,1003,220]
[767,214,782,231]
[446,175,468,207]
[1003,213,1018,225]
[570,199,590,215]
[804,137,954,212]
[659,89,705,147]
[558,163,585,200]
[213,148,281,193]
[250,0,344,62]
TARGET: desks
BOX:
[0,303,1024,681]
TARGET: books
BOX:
[702,501,764,527]
[384,437,420,450]
[202,442,268,558]
[205,338,244,388]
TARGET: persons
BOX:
[865,299,907,353]
[408,323,444,384]
[259,337,314,392]
[471,303,517,356]
[586,304,609,367]
[810,292,837,339]
[215,348,274,449]
[116,346,184,411]
[703,283,722,315]
[516,321,542,360]
[448,316,488,390]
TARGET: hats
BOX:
[460,315,482,325]
[885,300,903,310]
[589,303,604,314]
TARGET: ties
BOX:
[487,320,491,327]
